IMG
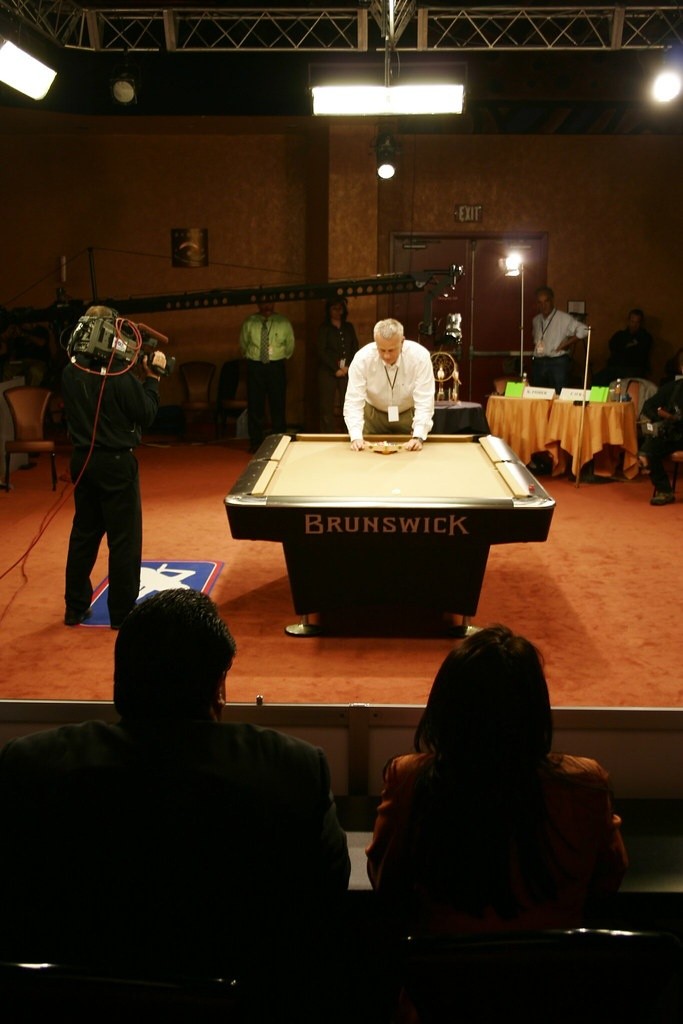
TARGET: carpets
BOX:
[76,557,223,628]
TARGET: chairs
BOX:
[610,378,659,438]
[627,381,639,421]
[222,360,247,437]
[3,386,57,492]
[653,451,683,498]
[0,962,244,1024]
[179,361,216,442]
[393,924,680,1024]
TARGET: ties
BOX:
[260,318,269,365]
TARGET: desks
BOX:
[223,432,556,638]
[485,394,639,484]
[429,400,485,434]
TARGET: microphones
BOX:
[138,323,170,343]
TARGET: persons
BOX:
[239,301,296,454]
[608,309,654,386]
[343,318,435,454]
[644,374,683,505]
[0,588,352,1024]
[527,286,589,395]
[367,621,630,1024]
[0,306,52,389]
[61,305,167,630]
[310,295,359,435]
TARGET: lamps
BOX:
[306,60,468,116]
[369,131,396,179]
[0,36,57,101]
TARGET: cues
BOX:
[575,324,592,489]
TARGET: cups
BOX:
[610,389,616,401]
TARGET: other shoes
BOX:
[248,447,255,454]
[111,607,136,629]
[63,606,93,625]
[651,492,675,505]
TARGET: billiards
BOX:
[371,441,399,455]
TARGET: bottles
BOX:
[615,379,622,402]
[523,372,528,387]
